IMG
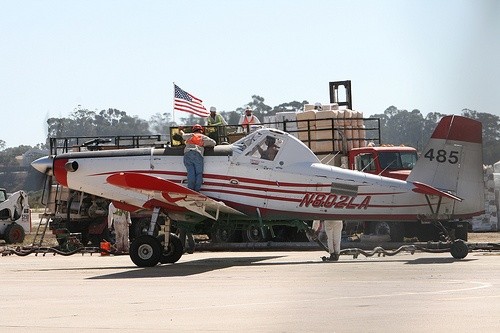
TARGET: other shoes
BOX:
[115,251,121,254]
[327,256,337,260]
[335,255,339,260]
[125,251,129,253]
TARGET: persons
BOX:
[180,124,217,193]
[108,201,132,255]
[324,219,343,260]
[207,106,231,137]
[256,136,279,161]
[237,106,263,133]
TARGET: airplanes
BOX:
[31,114,487,269]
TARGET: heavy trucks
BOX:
[41,118,419,246]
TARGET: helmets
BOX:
[192,124,203,131]
[210,106,216,111]
[245,106,252,111]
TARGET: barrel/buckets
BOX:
[101,242,110,256]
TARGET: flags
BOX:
[174,84,210,118]
